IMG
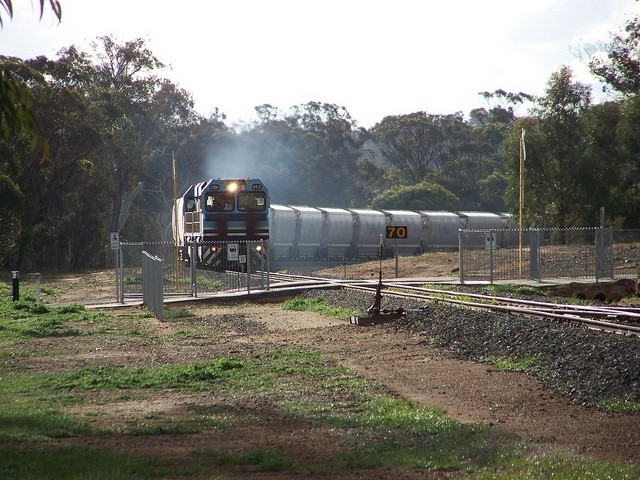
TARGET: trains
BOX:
[172,177,529,270]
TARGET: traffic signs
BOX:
[387,226,407,239]
